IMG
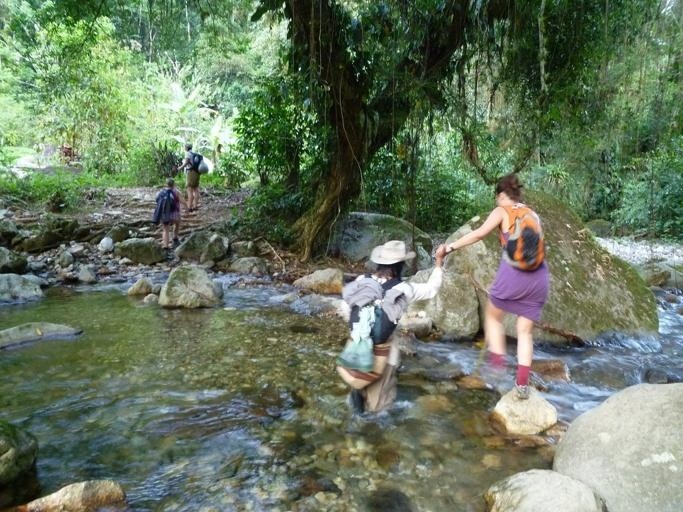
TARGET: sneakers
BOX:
[514,380,531,399]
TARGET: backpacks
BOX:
[501,203,545,271]
[189,152,202,170]
[161,190,176,212]
[349,274,402,345]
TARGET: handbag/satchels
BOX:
[337,344,390,389]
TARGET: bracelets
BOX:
[449,243,454,251]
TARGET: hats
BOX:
[370,240,416,265]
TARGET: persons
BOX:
[344,240,447,417]
[435,174,551,401]
[155,179,191,250]
[171,143,200,212]
